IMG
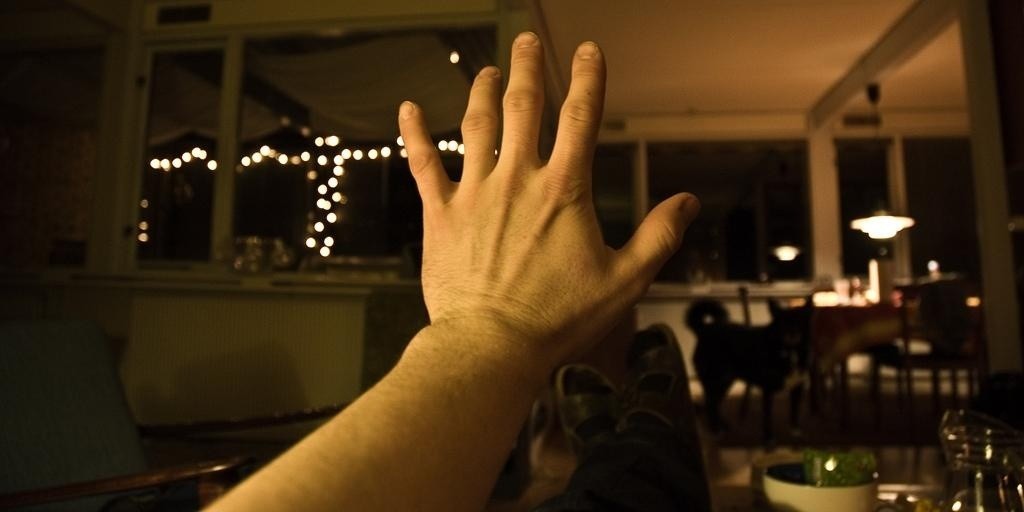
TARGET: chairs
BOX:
[0,306,255,512]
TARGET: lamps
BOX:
[850,80,916,240]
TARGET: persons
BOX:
[202,31,703,511]
